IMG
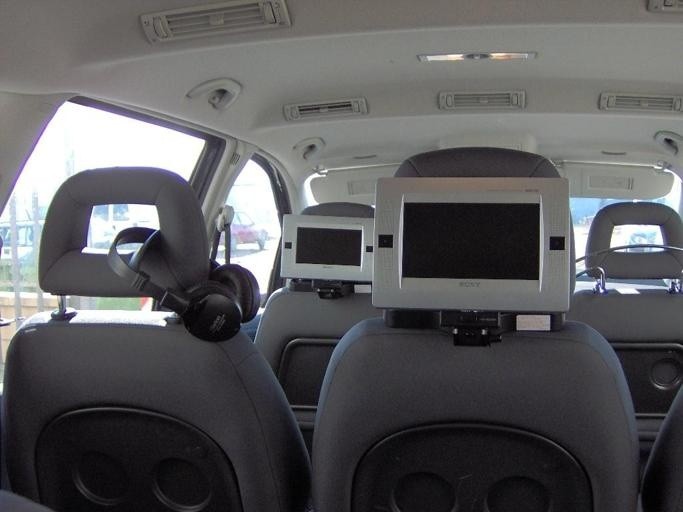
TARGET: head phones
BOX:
[106,226,260,343]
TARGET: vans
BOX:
[90,204,134,249]
[0,221,45,261]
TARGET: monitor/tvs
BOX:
[371,176,571,312]
[279,213,374,282]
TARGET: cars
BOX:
[220,206,269,252]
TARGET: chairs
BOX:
[2,166,312,511]
[252,201,384,463]
[313,147,639,511]
[566,201,683,475]
[638,384,682,511]
